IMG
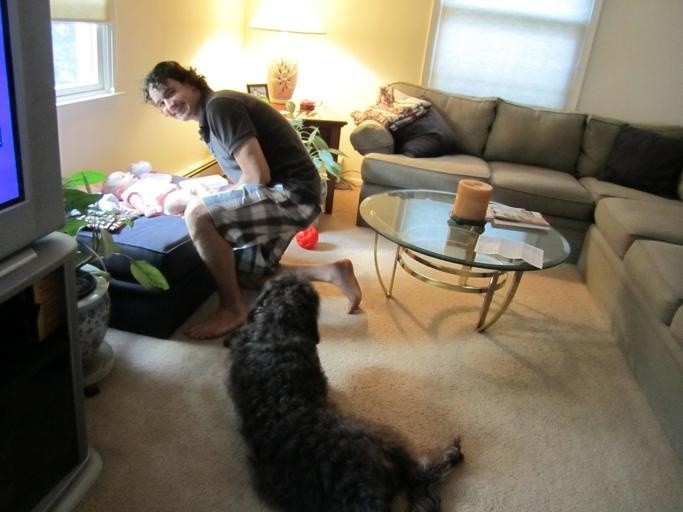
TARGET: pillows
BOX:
[481,97,588,174]
[383,82,499,158]
[598,122,683,199]
[396,95,458,158]
[573,114,683,177]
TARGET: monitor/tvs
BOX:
[0,0,65,279]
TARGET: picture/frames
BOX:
[246,84,271,104]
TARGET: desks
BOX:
[302,119,349,215]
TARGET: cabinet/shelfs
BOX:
[0,230,104,512]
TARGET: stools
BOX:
[75,172,244,339]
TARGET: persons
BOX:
[101,170,200,218]
[141,61,362,340]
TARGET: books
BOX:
[490,204,551,232]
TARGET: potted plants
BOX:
[58,172,172,399]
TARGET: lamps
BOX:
[247,0,328,111]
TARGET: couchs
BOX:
[349,81,683,463]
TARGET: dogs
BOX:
[223,273,464,512]
